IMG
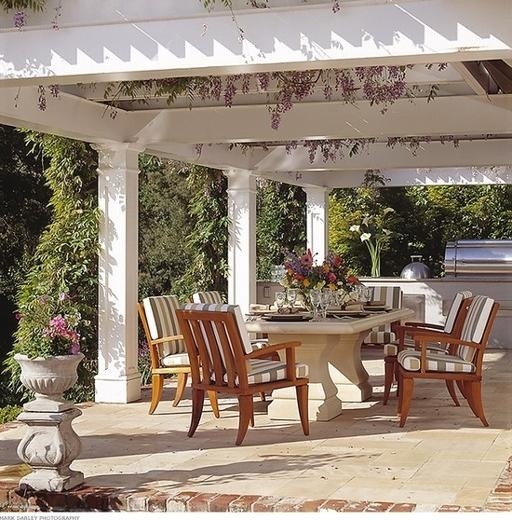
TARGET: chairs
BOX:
[245,283,501,429]
[139,288,311,447]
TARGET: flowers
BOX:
[275,250,364,295]
[349,207,396,276]
[14,292,84,357]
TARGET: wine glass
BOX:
[274,289,373,319]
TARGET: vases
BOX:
[365,240,381,278]
[288,287,362,322]
[14,351,84,413]
